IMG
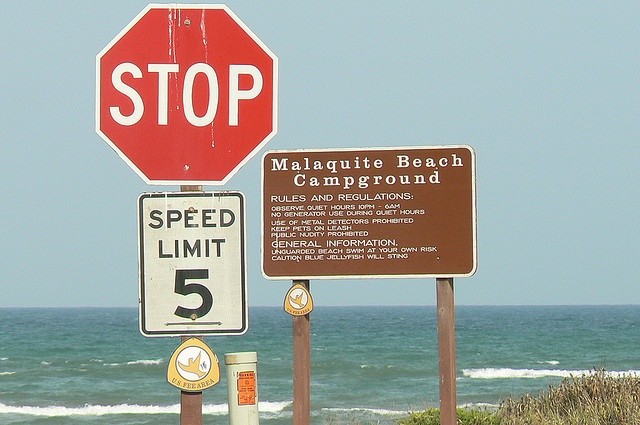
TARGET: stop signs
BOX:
[94,4,277,185]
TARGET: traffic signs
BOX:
[135,189,250,338]
